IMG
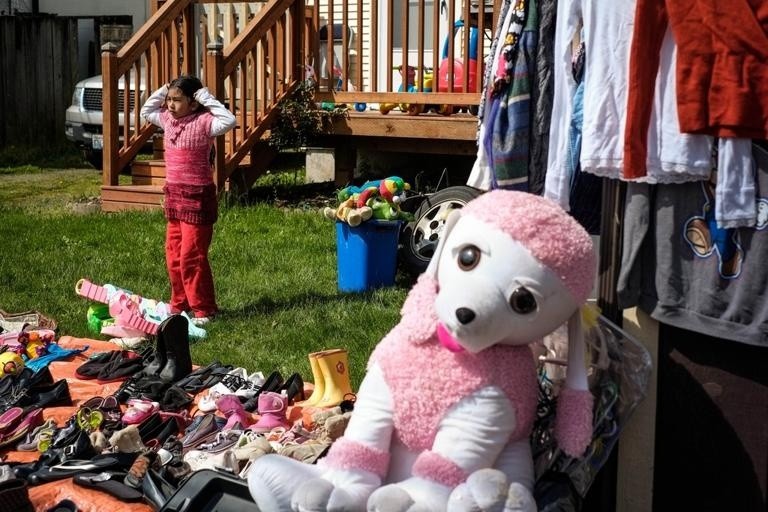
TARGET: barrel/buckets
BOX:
[336,218,398,292]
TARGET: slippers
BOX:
[73,472,143,503]
[3,406,43,446]
[0,407,25,442]
[28,456,119,485]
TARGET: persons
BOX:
[139,77,240,326]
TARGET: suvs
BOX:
[65,67,151,169]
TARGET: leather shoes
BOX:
[142,466,179,509]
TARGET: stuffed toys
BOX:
[323,176,417,226]
[251,188,597,511]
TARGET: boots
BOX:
[109,427,173,466]
[297,349,341,407]
[315,349,354,407]
[216,394,253,431]
[250,391,288,432]
[160,315,193,383]
[143,315,177,376]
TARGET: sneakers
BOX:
[137,413,179,447]
[277,408,352,465]
[74,348,128,380]
[123,453,162,488]
[97,352,144,385]
[55,394,123,447]
[191,316,215,326]
[175,422,278,477]
[44,499,83,511]
[17,418,59,452]
[117,379,195,412]
[176,361,234,394]
[0,366,74,416]
[231,371,266,409]
[122,398,160,424]
[198,367,248,412]
[0,479,37,512]
[182,414,220,448]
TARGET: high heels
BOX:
[276,371,306,407]
[243,371,283,412]
